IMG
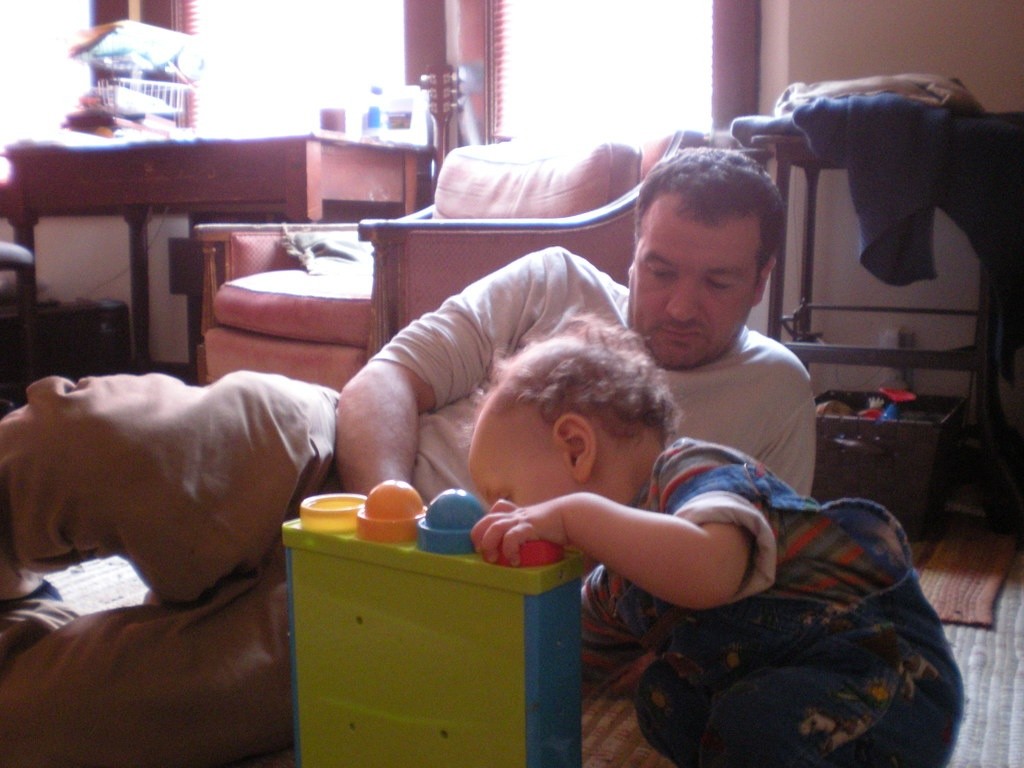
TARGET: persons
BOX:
[0,147,816,768]
[466,312,965,768]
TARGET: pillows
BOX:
[279,224,374,278]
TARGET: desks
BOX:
[0,128,432,389]
[730,116,1024,554]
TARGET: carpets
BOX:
[910,483,1016,630]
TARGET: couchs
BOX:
[188,127,714,393]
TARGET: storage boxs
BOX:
[811,387,968,543]
